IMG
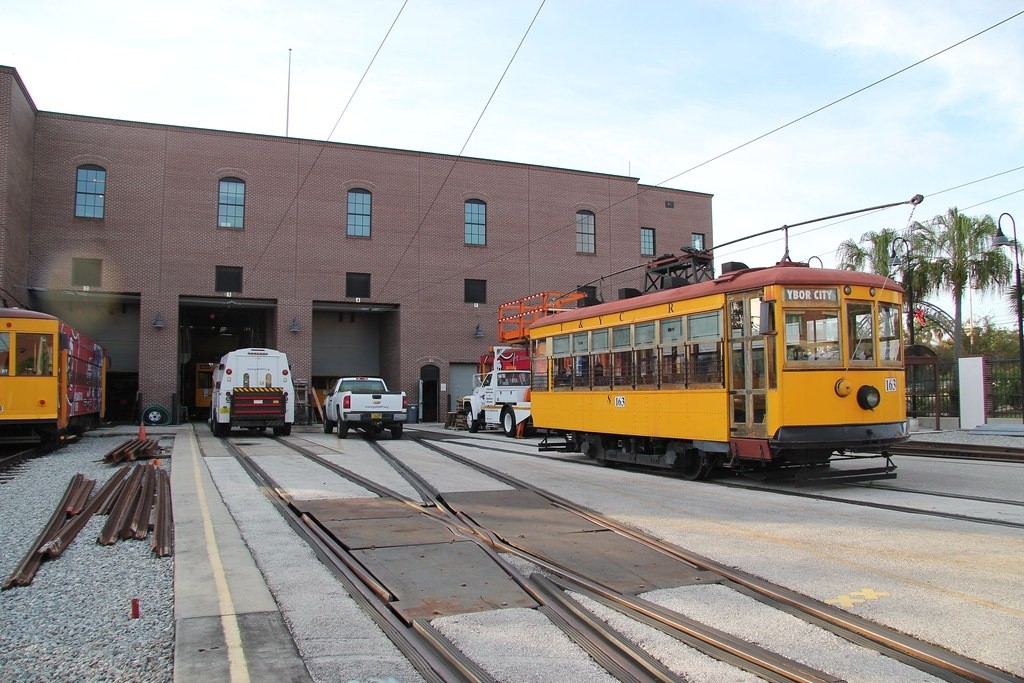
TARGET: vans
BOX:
[209,347,296,438]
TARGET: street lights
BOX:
[992,212,1024,426]
[886,237,917,418]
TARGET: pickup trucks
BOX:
[321,377,408,440]
[462,369,533,437]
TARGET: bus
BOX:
[194,359,219,412]
[0,305,112,445]
[528,235,909,487]
